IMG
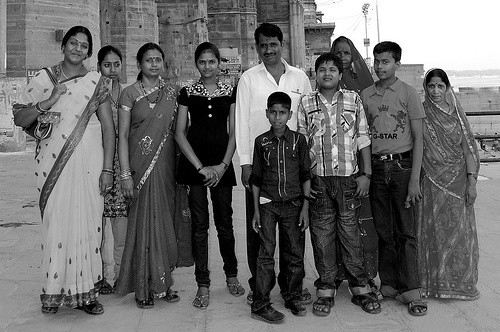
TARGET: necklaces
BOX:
[60,64,67,79]
[139,79,161,110]
[111,87,120,105]
[445,101,450,114]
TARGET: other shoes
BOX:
[370,287,384,300]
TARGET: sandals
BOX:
[41,305,58,314]
[312,297,335,316]
[300,288,313,304]
[83,299,105,315]
[226,277,245,297]
[351,291,381,314]
[194,294,209,309]
[135,295,154,309]
[408,299,428,316]
[246,290,254,305]
[161,288,180,303]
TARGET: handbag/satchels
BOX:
[22,67,53,140]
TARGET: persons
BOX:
[12,25,116,314]
[235,22,313,303]
[178,42,245,308]
[97,45,126,294]
[362,42,427,315]
[117,43,194,309]
[247,93,313,324]
[337,37,384,300]
[422,67,480,300]
[297,52,381,317]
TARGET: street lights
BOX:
[361,2,373,72]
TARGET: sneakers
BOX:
[251,302,286,323]
[285,298,307,316]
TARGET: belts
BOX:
[372,148,413,160]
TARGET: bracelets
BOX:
[222,161,230,169]
[301,194,311,202]
[466,172,478,182]
[363,172,373,179]
[101,169,114,175]
[120,169,131,180]
[196,166,204,172]
[36,102,48,113]
